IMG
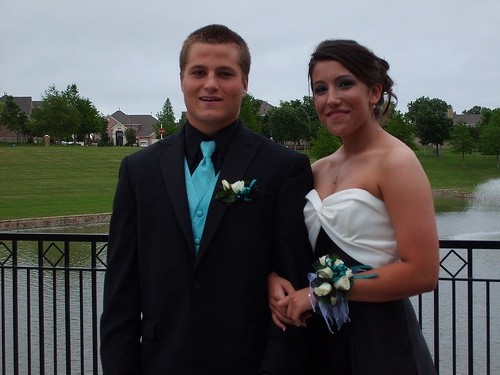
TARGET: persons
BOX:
[267,38,441,375]
[99,23,316,375]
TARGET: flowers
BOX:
[307,254,356,303]
[213,179,258,208]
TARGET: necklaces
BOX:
[331,125,381,184]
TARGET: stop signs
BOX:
[158,128,165,135]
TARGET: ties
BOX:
[191,139,218,204]
